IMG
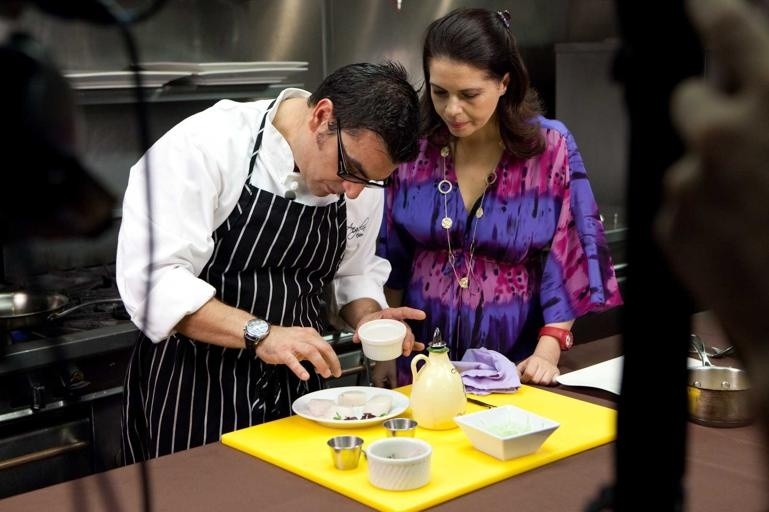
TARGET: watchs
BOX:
[243,318,271,355]
[538,327,574,352]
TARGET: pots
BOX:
[2,290,124,337]
[686,332,754,429]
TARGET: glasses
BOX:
[323,95,392,190]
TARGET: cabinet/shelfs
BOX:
[0,309,769,512]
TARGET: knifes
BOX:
[709,343,737,360]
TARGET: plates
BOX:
[291,385,408,427]
[555,352,623,399]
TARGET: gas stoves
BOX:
[0,266,131,441]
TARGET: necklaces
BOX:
[438,140,505,289]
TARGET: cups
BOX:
[383,417,418,437]
[326,436,364,470]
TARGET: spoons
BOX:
[690,341,737,361]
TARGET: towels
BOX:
[449,346,522,395]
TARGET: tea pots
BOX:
[408,330,467,431]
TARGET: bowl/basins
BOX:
[357,318,409,361]
[455,403,559,460]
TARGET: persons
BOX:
[115,61,427,467]
[371,9,625,389]
[655,0,769,464]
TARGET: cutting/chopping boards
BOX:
[221,376,618,510]
